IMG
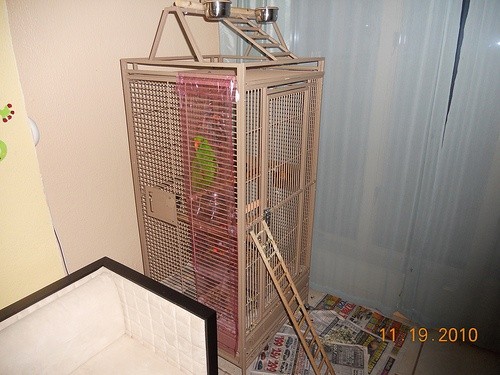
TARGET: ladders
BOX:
[248,220,336,375]
